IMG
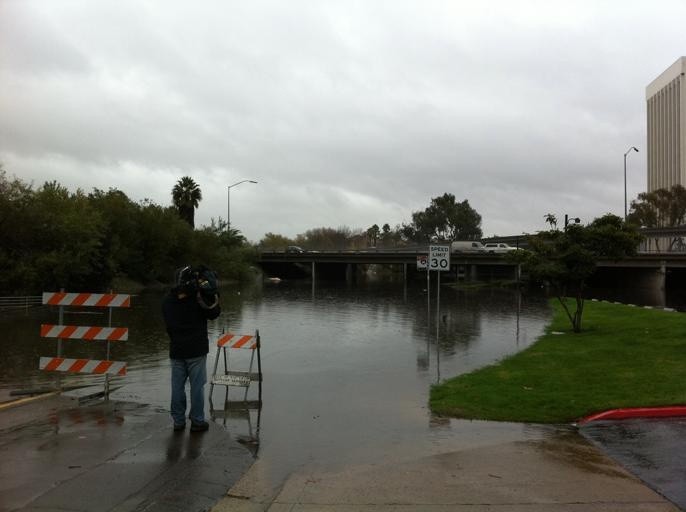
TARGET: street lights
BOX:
[228,179,258,232]
[565,215,580,233]
[624,146,639,222]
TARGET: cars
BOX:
[484,243,524,256]
[285,245,304,254]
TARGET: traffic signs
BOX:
[429,244,449,272]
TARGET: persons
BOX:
[160,266,221,434]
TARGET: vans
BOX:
[451,241,488,255]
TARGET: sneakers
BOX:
[173,422,186,433]
[189,421,209,433]
